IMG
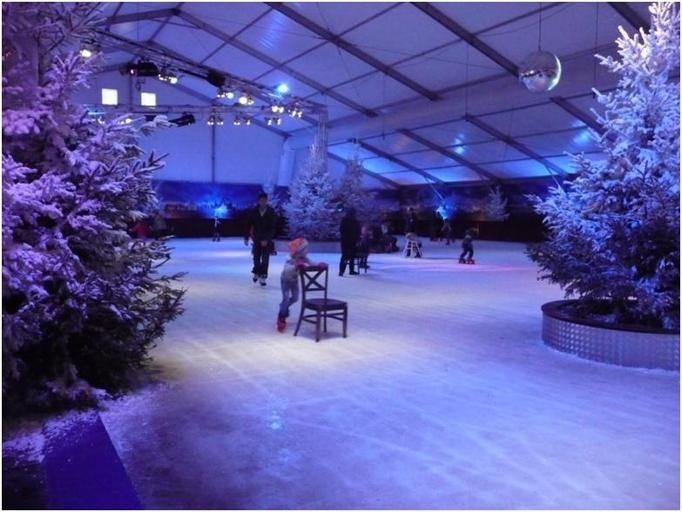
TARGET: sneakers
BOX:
[277,316,285,329]
[350,272,359,274]
[253,273,259,282]
[339,272,343,276]
[260,278,266,286]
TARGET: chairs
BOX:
[293,267,347,342]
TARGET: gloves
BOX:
[295,262,305,269]
[318,262,325,270]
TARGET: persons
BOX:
[429,210,444,241]
[128,221,153,244]
[212,216,222,242]
[243,191,278,286]
[408,207,419,234]
[361,218,399,255]
[277,237,326,331]
[458,227,481,261]
[436,211,443,221]
[338,207,362,275]
[440,218,456,245]
[405,232,423,257]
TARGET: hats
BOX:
[289,238,309,257]
